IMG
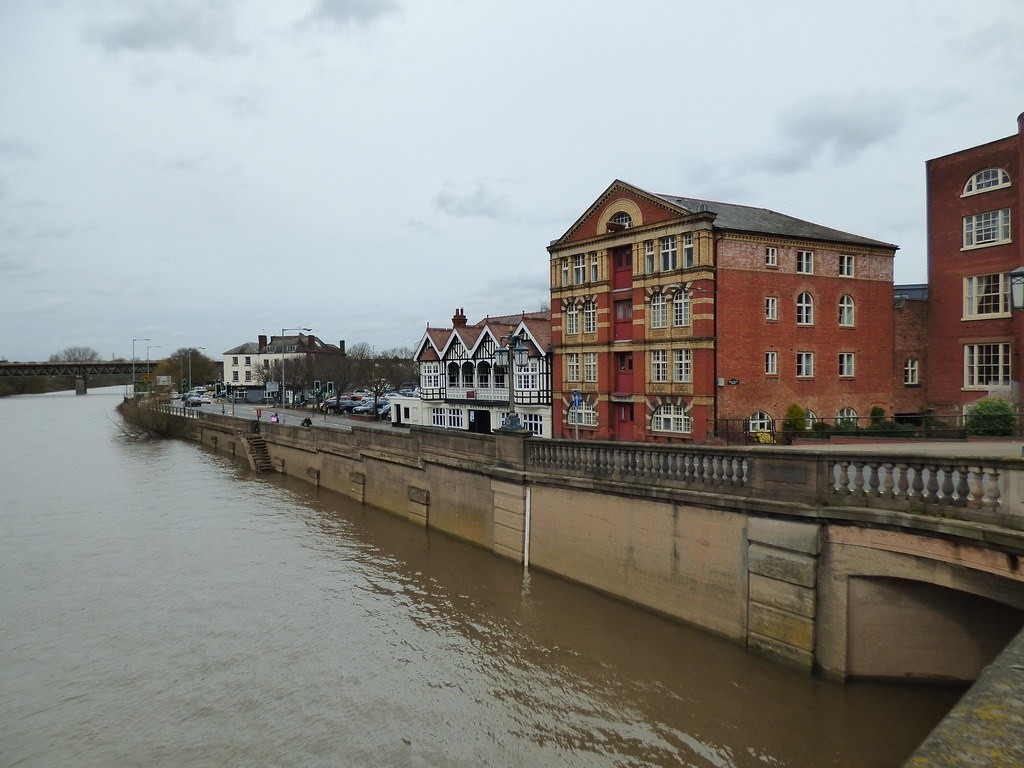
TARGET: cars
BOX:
[319,386,420,416]
[158,393,172,405]
[169,387,211,408]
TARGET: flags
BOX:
[573,390,581,407]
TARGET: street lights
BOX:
[147,345,164,387]
[189,345,205,409]
[281,328,313,424]
[503,332,531,430]
[132,337,150,387]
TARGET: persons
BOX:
[274,413,279,423]
[302,417,312,427]
